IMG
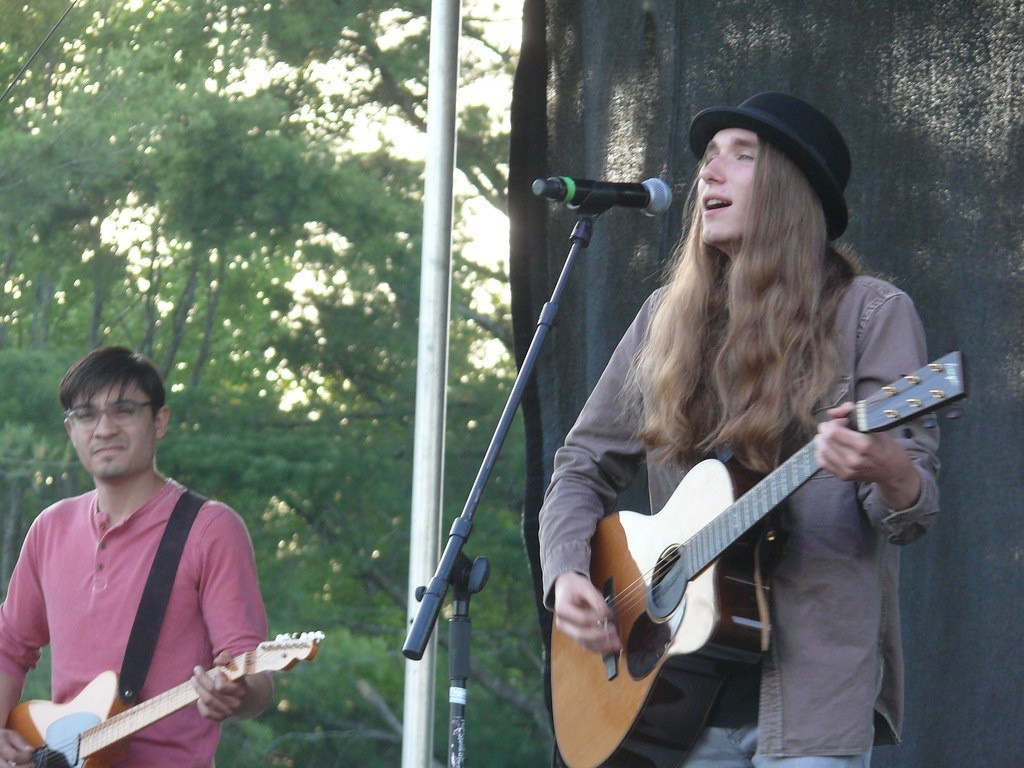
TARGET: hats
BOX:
[686,91,850,241]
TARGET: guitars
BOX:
[552,350,967,768]
[8,627,326,768]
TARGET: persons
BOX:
[0,345,275,768]
[537,92,939,768]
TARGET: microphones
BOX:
[532,175,674,217]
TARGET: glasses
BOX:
[62,401,150,431]
